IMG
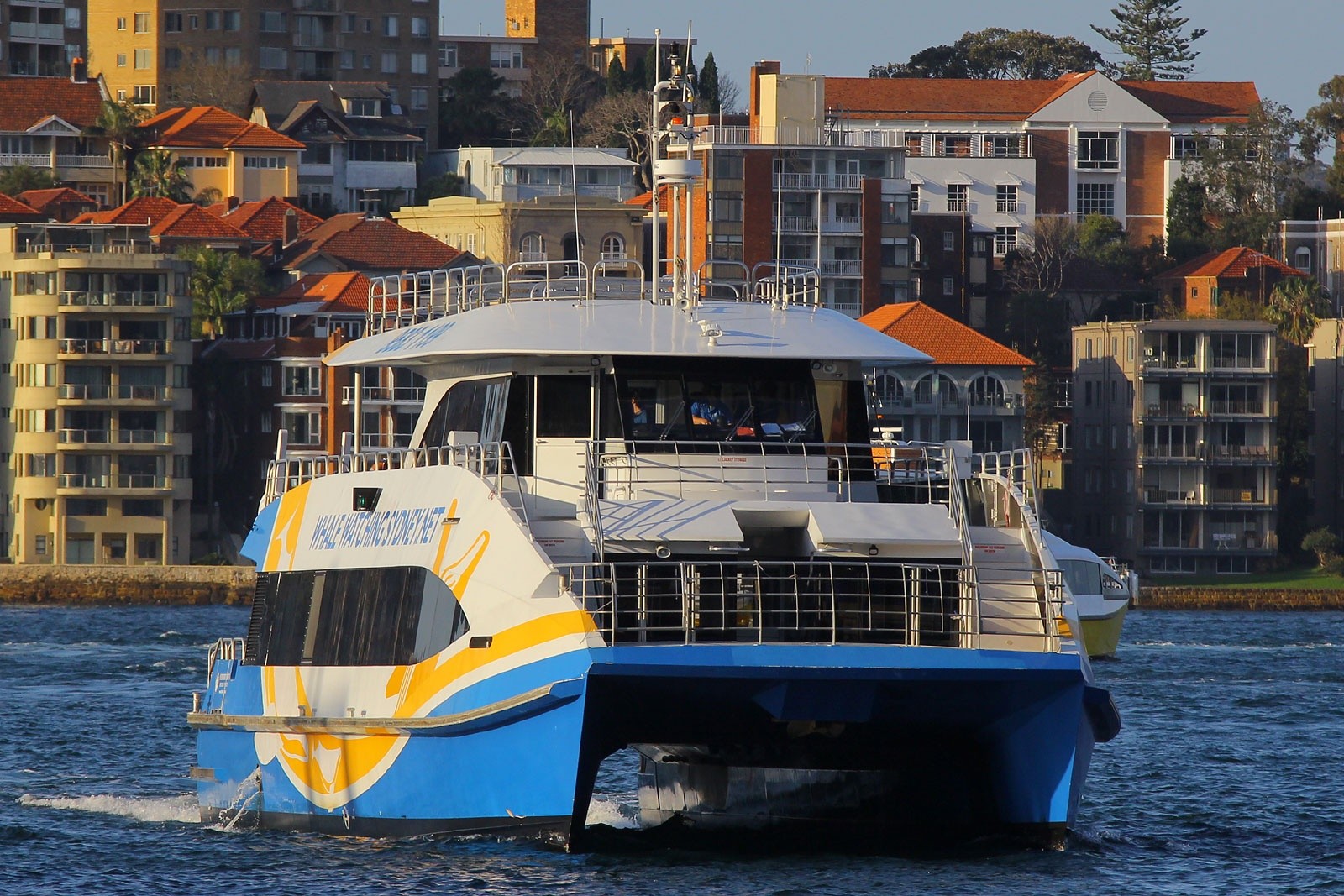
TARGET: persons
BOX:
[691,382,733,426]
[604,555,962,646]
[631,390,649,440]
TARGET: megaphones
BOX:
[823,364,837,373]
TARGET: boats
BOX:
[1040,528,1140,658]
[185,13,1120,864]
